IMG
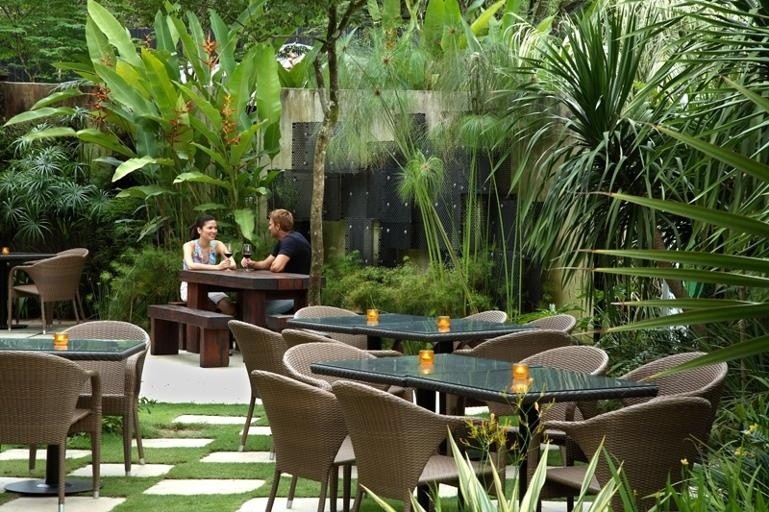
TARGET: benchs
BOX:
[168,301,239,350]
[147,305,234,367]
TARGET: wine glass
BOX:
[241,244,253,272]
[223,242,234,272]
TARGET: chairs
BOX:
[7,254,84,334]
[0,350,102,512]
[27,320,152,477]
[14,248,89,328]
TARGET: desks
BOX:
[179,270,310,363]
[0,254,58,329]
[0,337,147,496]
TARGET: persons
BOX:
[180,213,237,315]
[241,209,311,315]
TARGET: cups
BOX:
[54,333,69,349]
[437,316,451,328]
[367,308,378,321]
[419,350,434,365]
[512,363,528,379]
